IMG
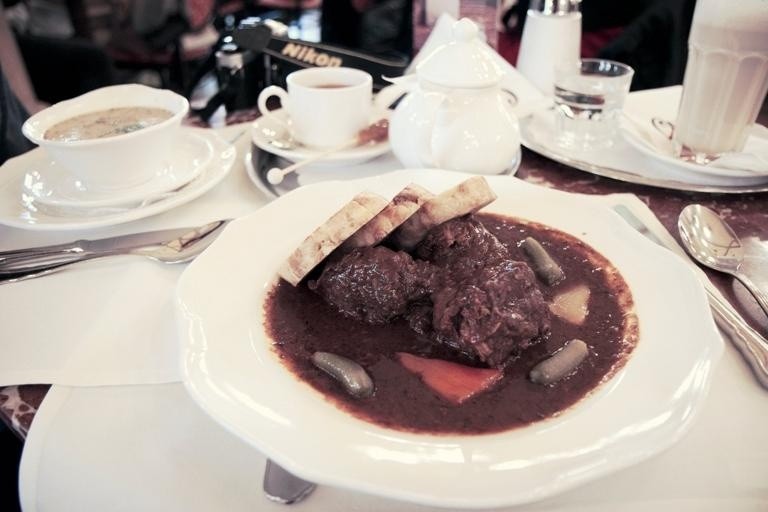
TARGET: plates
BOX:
[623,115,767,178]
[14,279,768,512]
[517,92,767,198]
[0,126,237,231]
[174,167,725,510]
[23,140,202,207]
[251,107,392,160]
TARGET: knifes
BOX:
[614,203,768,390]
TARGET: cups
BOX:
[258,66,372,149]
[551,57,635,166]
[671,0,768,166]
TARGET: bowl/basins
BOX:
[20,83,190,193]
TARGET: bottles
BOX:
[516,0,585,92]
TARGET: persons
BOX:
[0,0,50,149]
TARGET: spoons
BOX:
[676,205,768,325]
[6,216,233,272]
